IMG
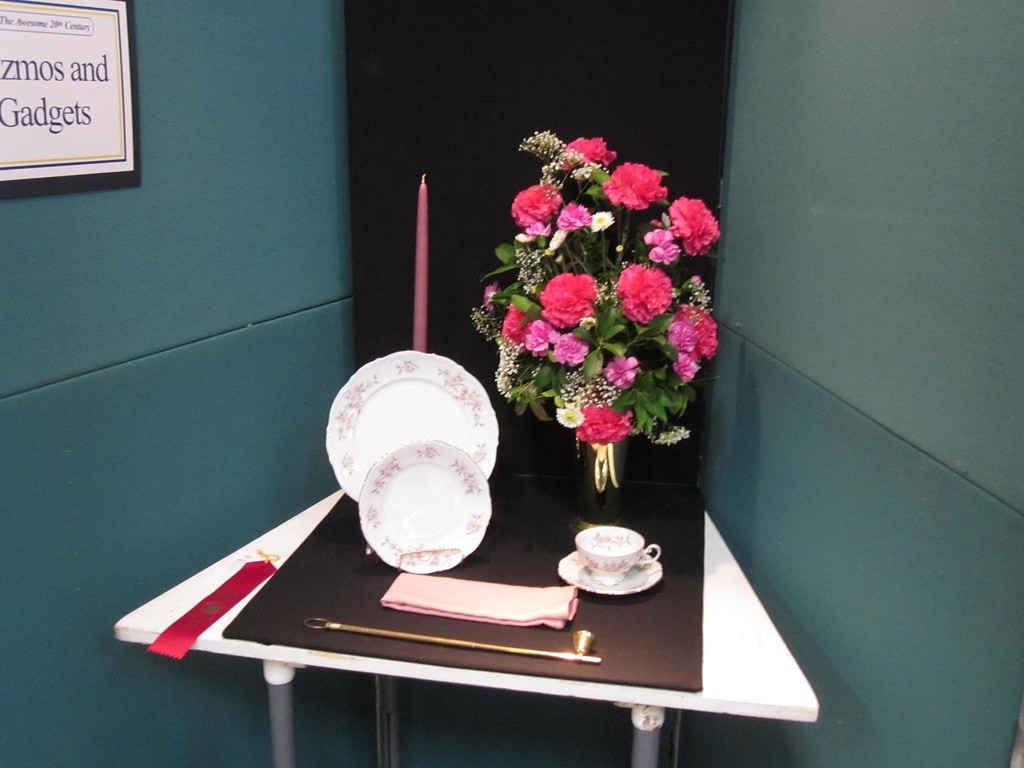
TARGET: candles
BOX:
[412,173,429,351]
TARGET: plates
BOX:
[359,441,493,575]
[324,350,500,503]
[557,550,663,595]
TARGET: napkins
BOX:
[380,572,580,630]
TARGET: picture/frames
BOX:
[0,0,145,192]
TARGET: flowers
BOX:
[469,130,719,445]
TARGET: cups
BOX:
[575,525,661,587]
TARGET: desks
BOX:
[114,487,819,768]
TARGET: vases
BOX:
[568,436,629,534]
